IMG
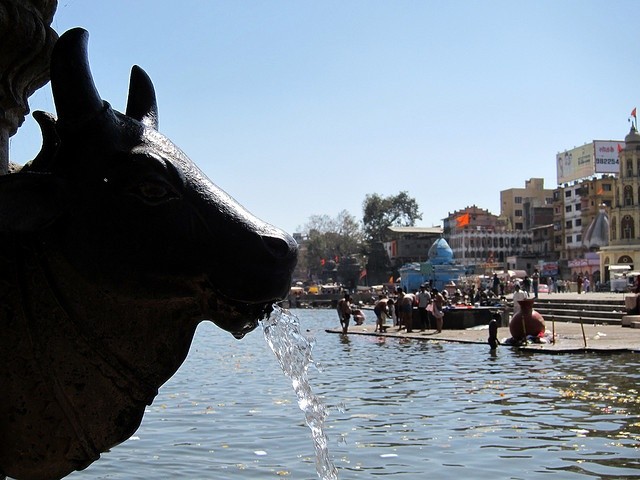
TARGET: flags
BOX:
[455,214,469,229]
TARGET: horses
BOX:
[1,24,304,480]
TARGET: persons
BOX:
[502,277,520,293]
[373,298,393,334]
[337,293,354,334]
[468,274,502,305]
[577,274,591,294]
[520,269,540,298]
[416,281,433,333]
[513,285,526,314]
[352,309,366,326]
[440,286,469,306]
[402,292,417,333]
[545,274,572,294]
[488,311,502,352]
[393,286,405,326]
[431,287,446,334]
